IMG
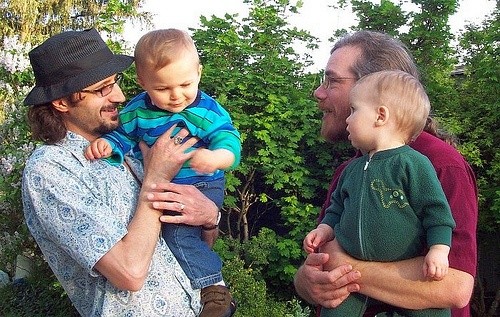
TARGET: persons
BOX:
[293,31,478,317]
[22,28,242,317]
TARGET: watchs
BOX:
[203,211,221,231]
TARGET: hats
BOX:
[24,26,136,106]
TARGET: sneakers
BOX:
[199,285,236,317]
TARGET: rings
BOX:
[173,135,183,144]
[179,203,184,211]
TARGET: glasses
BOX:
[320,74,358,89]
[79,72,124,97]
